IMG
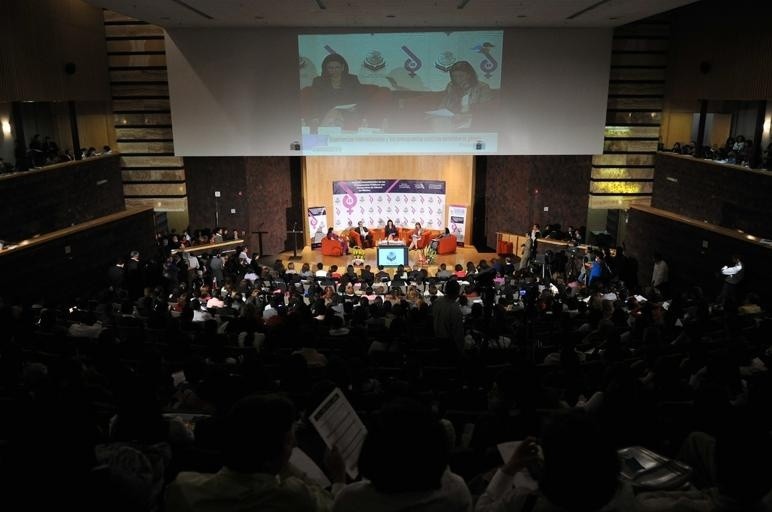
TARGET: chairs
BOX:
[389,90,499,134]
[320,234,347,257]
[380,225,403,242]
[0,253,772,512]
[404,228,432,249]
[299,82,394,133]
[429,232,457,256]
[349,226,377,251]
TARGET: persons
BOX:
[430,59,496,132]
[386,67,432,131]
[307,53,370,128]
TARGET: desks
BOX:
[378,238,404,245]
[302,123,385,146]
